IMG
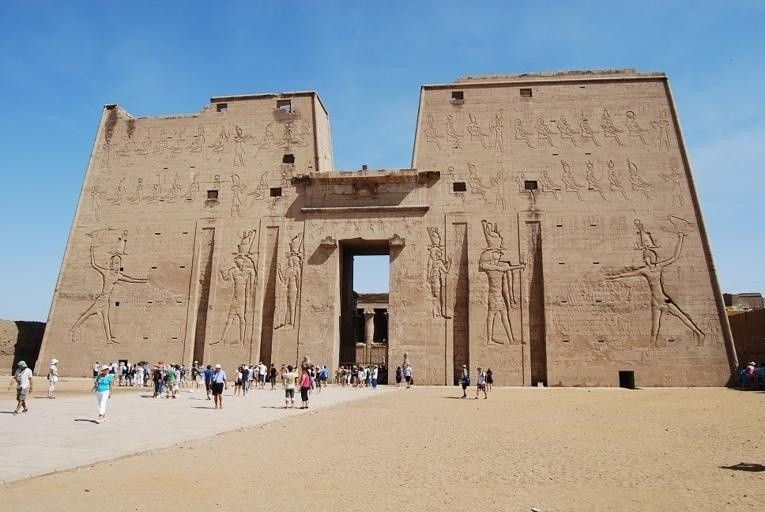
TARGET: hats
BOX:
[119,356,379,367]
[101,365,110,372]
[18,360,28,368]
[49,358,59,365]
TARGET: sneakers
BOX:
[232,384,275,398]
[48,395,56,399]
[96,417,107,424]
[118,383,211,400]
[310,383,376,395]
[11,409,28,416]
[284,405,308,409]
[461,393,488,401]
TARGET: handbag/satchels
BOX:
[464,376,470,386]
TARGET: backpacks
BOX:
[321,372,325,380]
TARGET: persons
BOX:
[94,365,112,423]
[476,366,488,400]
[46,358,60,399]
[7,360,33,416]
[743,361,756,388]
[461,364,469,399]
[485,367,494,391]
[756,360,765,388]
